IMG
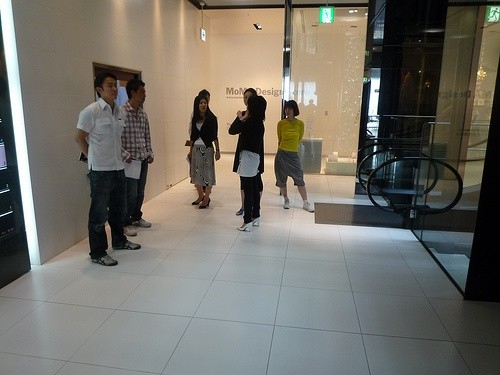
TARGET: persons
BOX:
[186,88,267,232]
[76,73,141,266]
[119,79,154,235]
[274,100,315,213]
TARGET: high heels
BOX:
[237,217,261,232]
[192,195,211,209]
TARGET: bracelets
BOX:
[216,148,219,152]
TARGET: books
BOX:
[77,144,89,162]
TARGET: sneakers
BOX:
[132,218,151,228]
[303,202,314,212]
[123,226,137,236]
[112,241,141,250]
[284,201,290,209]
[91,254,118,266]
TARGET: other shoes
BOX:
[236,208,243,215]
